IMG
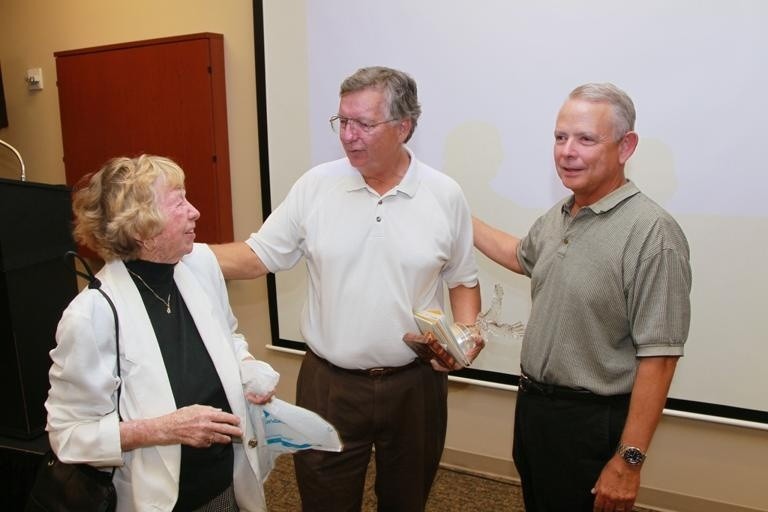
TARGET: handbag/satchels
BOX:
[24,452,117,511]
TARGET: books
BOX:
[415,308,471,369]
[402,331,459,371]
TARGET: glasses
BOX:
[329,115,400,134]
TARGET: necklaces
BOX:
[127,268,173,316]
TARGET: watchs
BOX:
[616,441,648,466]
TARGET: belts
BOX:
[518,369,600,400]
[305,346,424,376]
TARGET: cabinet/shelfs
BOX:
[53,33,233,243]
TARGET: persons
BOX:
[469,80,698,511]
[209,63,488,511]
[43,153,286,512]
